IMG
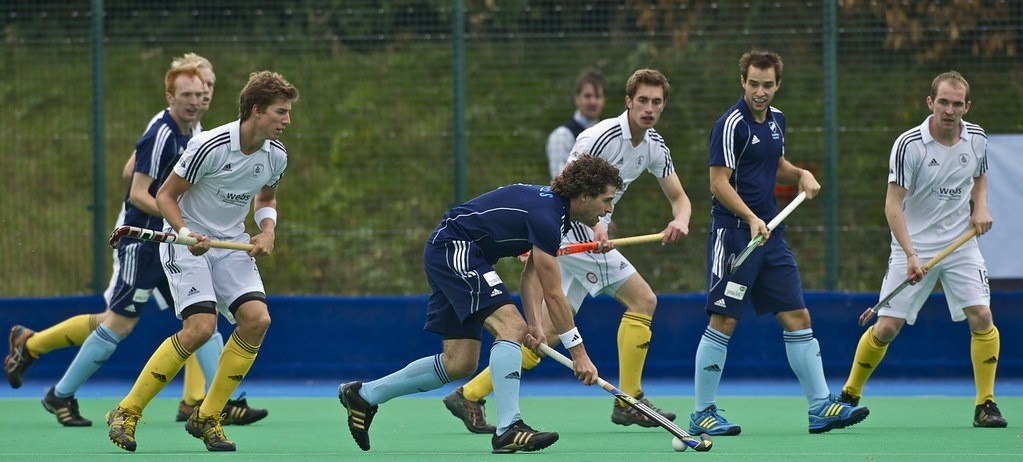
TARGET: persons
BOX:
[38,65,269,428]
[689,51,869,436]
[105,71,300,453]
[4,51,218,422]
[841,71,1011,432]
[335,155,626,451]
[0,1,1023,72]
[543,69,607,185]
[443,67,692,434]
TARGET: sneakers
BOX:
[198,392,268,425]
[808,393,870,433]
[688,405,741,436]
[105,405,145,452]
[338,381,378,451]
[5,325,39,387]
[836,390,860,407]
[610,391,677,428]
[176,400,197,421]
[41,387,91,426]
[185,407,236,452]
[442,386,496,434]
[973,400,1007,427]
[491,420,559,453]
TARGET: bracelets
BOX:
[255,206,278,228]
[560,324,583,347]
[179,226,190,236]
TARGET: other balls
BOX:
[672,436,688,451]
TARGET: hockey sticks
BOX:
[109,227,254,252]
[725,190,807,277]
[858,227,978,327]
[523,334,713,451]
[517,232,665,264]
